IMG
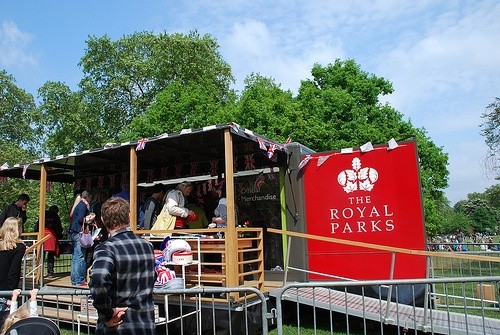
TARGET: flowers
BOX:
[241,221,249,233]
[208,217,225,228]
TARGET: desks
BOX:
[174,237,252,286]
[161,261,199,289]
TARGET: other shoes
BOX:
[79,282,89,287]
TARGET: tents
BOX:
[0,121,290,287]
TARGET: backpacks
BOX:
[137,197,156,229]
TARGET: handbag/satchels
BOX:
[92,220,103,241]
[79,218,94,248]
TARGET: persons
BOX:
[92,182,239,238]
[426,227,496,251]
[68,190,92,286]
[33,205,63,278]
[0,194,30,233]
[88,197,156,335]
[0,216,26,309]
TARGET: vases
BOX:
[214,234,219,239]
[239,232,242,237]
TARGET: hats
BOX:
[214,181,226,190]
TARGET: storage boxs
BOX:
[172,251,194,263]
